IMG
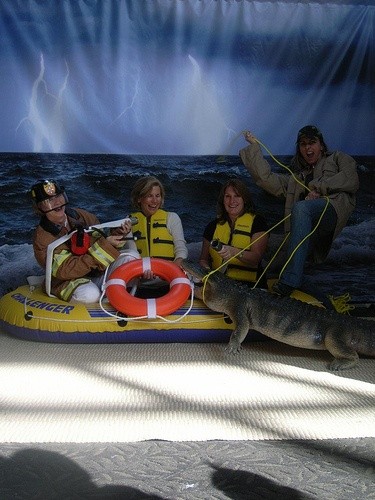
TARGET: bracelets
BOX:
[235,248,245,259]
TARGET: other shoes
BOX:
[271,281,295,297]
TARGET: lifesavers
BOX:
[106,258,191,317]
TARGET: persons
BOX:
[27,177,132,303]
[124,175,188,281]
[240,125,359,295]
[199,180,271,290]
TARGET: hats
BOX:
[296,125,322,143]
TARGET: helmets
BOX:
[31,178,70,214]
